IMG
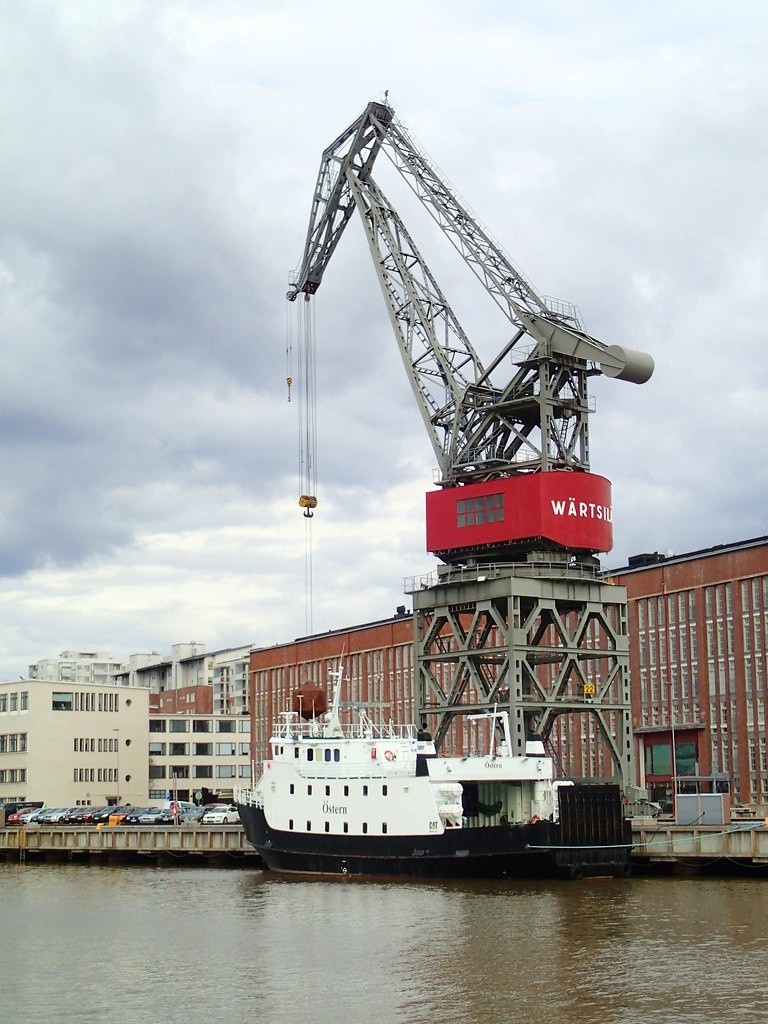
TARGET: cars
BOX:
[0,801,241,825]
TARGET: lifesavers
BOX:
[500,816,508,826]
[385,750,394,761]
[171,806,177,815]
[531,815,540,824]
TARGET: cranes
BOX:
[282,100,656,821]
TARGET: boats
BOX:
[231,639,637,882]
